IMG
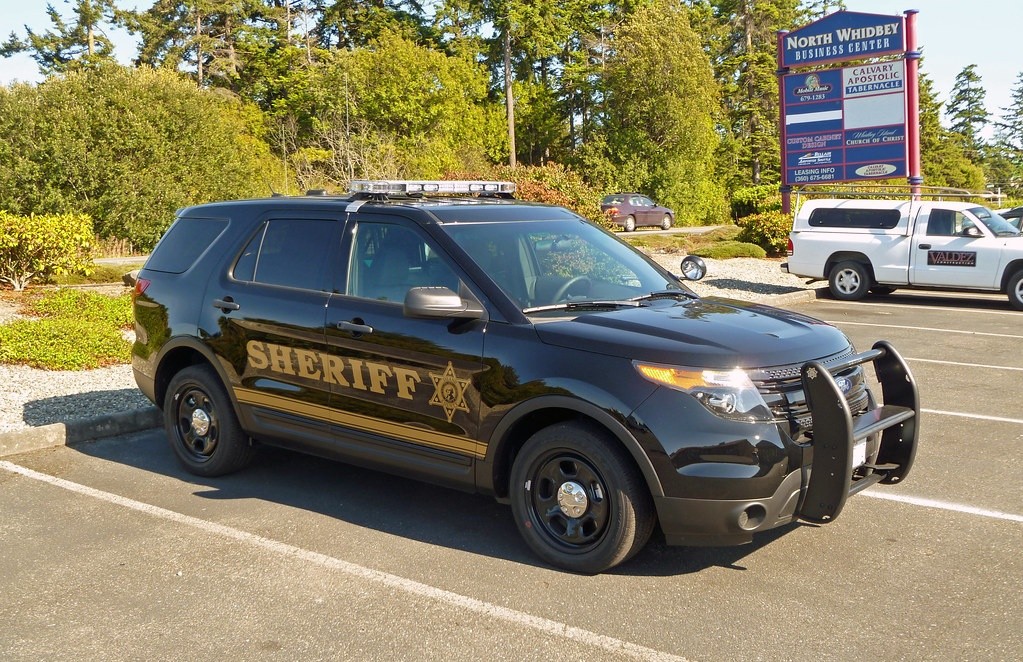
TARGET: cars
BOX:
[961,206,1023,235]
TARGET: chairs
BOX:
[369,246,412,304]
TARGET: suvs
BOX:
[779,199,1023,309]
[129,178,922,576]
[602,192,676,232]
